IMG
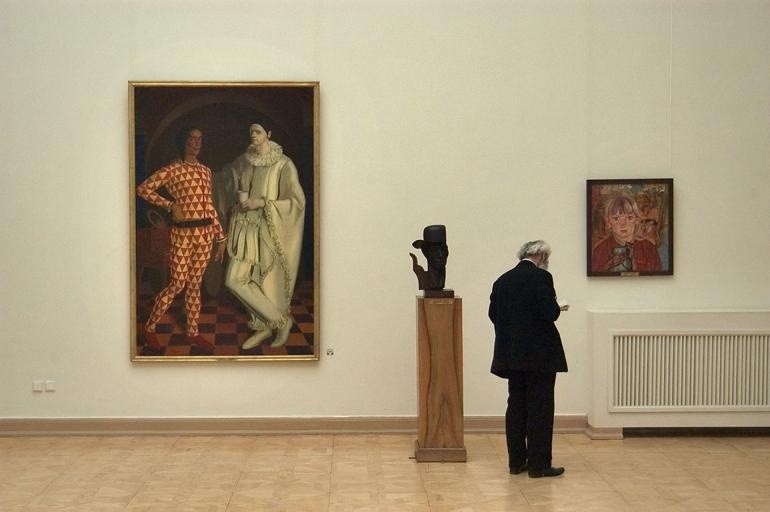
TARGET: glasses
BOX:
[610,214,637,224]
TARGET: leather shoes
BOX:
[528,467,565,478]
[509,458,528,474]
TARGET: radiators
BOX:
[585,308,770,430]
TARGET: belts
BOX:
[171,217,213,227]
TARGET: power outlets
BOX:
[45,381,56,392]
[32,380,43,392]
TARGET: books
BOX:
[555,299,568,312]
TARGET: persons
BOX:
[487,240,569,478]
[219,113,306,351]
[592,195,660,272]
[136,126,225,351]
[409,225,448,290]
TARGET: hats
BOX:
[412,225,446,248]
[176,126,200,162]
[248,111,280,137]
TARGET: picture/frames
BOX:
[585,177,675,279]
[128,80,322,364]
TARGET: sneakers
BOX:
[242,328,273,350]
[139,325,161,350]
[270,317,293,348]
[183,333,217,351]
